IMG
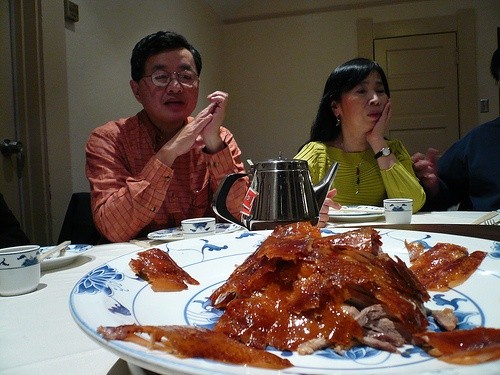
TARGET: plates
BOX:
[147,222,243,242]
[327,205,385,222]
[69,227,500,375]
[39,244,93,271]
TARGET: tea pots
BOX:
[212,151,342,231]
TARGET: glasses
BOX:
[140,69,201,87]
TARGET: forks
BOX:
[483,218,500,225]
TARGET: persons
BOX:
[412,46,500,211]
[85,30,249,242]
[292,58,425,229]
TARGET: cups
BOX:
[181,216,216,237]
[383,198,413,224]
[0,245,41,297]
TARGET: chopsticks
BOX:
[328,209,384,213]
[39,241,71,261]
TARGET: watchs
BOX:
[374,146,392,159]
